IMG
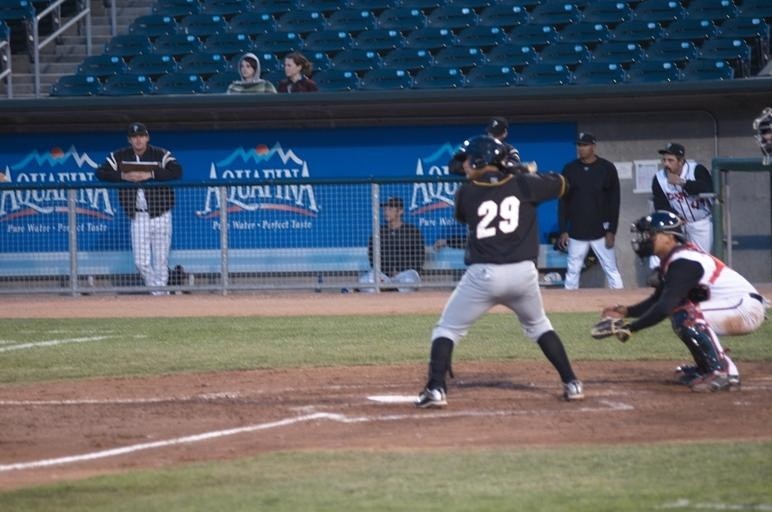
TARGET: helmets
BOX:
[752,106,772,166]
[631,210,685,257]
[454,135,506,169]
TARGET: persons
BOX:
[96,120,184,297]
[556,131,623,289]
[650,143,716,256]
[361,194,426,298]
[432,236,469,253]
[447,112,537,173]
[752,105,772,178]
[417,133,587,411]
[226,50,275,96]
[591,209,764,392]
[279,53,319,94]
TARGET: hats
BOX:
[127,122,147,136]
[484,117,507,136]
[574,132,595,144]
[658,142,685,156]
[380,196,403,208]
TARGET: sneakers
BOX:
[675,364,741,393]
[414,386,448,408]
[561,379,584,401]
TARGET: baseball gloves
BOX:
[591,317,632,343]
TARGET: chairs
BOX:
[1,0,76,86]
[50,1,772,96]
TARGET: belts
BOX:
[134,207,147,213]
[749,294,763,304]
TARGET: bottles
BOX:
[58,275,68,295]
[187,266,196,288]
[314,271,324,294]
[86,274,97,297]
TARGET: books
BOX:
[120,160,159,172]
